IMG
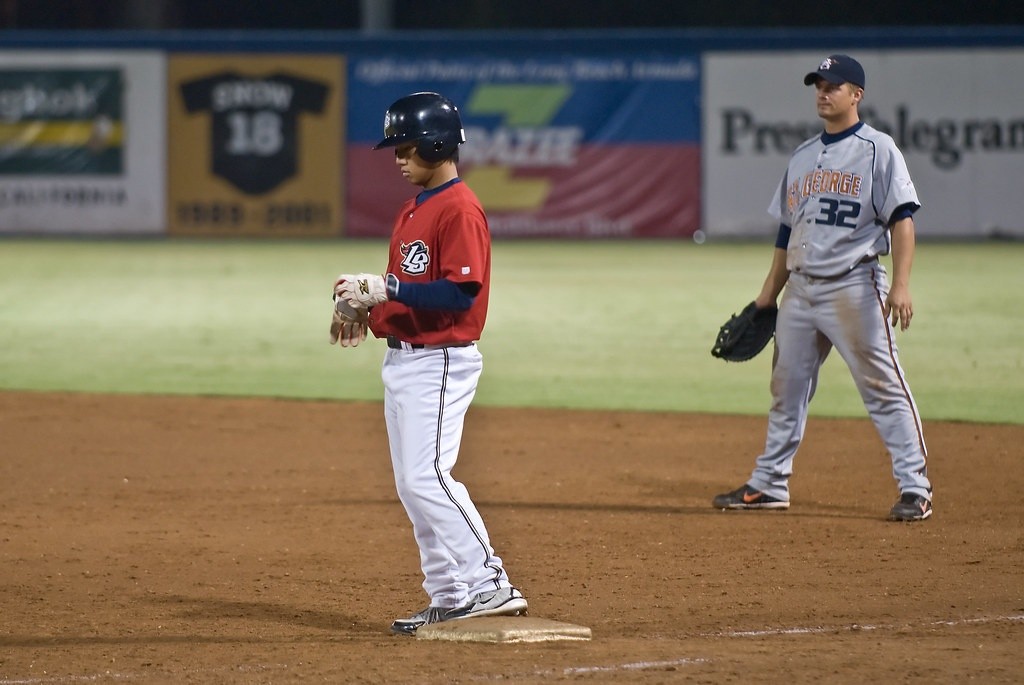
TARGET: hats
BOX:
[804,54,865,90]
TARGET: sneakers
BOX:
[889,492,932,521]
[390,606,455,635]
[445,587,529,621]
[712,484,790,510]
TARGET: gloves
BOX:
[333,273,388,310]
[329,295,368,347]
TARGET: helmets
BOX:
[370,92,466,162]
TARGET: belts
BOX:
[862,254,877,263]
[387,335,475,349]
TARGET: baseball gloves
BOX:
[711,300,778,363]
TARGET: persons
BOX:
[327,91,527,635]
[710,53,934,520]
